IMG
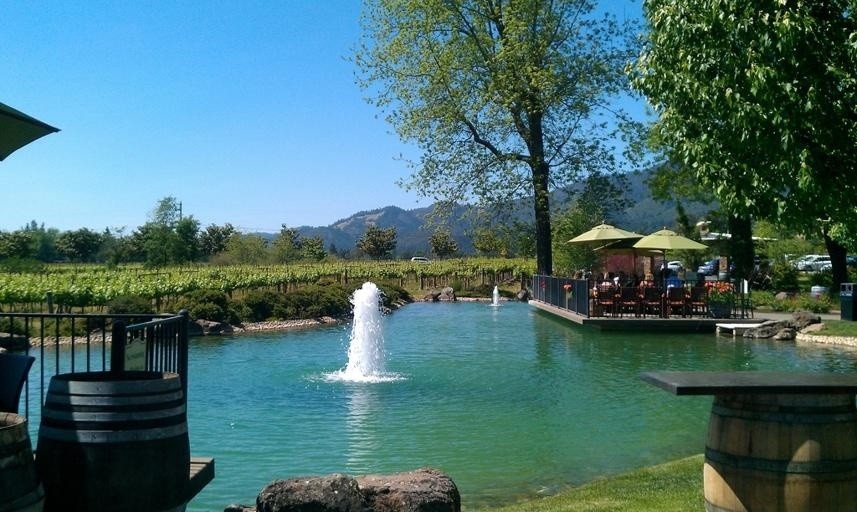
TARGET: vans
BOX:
[411,255,429,263]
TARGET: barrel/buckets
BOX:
[702,389,857,512]
[0,411,45,512]
[810,286,826,299]
[34,369,190,512]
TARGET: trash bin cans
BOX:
[840,283,857,321]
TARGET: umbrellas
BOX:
[565,219,649,282]
[591,230,664,284]
[606,225,710,299]
[0,102,62,162]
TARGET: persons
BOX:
[573,270,683,314]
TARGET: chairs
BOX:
[1,352,36,417]
[558,280,754,322]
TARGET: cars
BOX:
[659,253,856,289]
